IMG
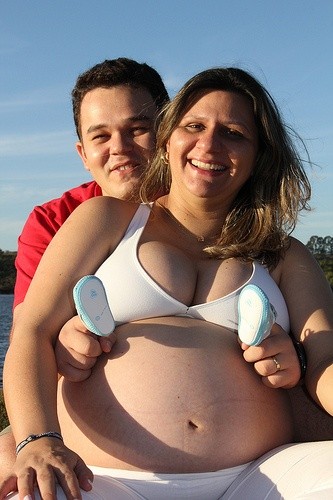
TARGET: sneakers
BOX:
[238,286,276,346]
[73,275,115,336]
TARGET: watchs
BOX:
[290,333,307,383]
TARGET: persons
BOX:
[1,67,333,499]
[11,57,310,389]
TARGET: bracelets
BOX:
[15,430,64,455]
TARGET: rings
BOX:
[273,356,281,371]
